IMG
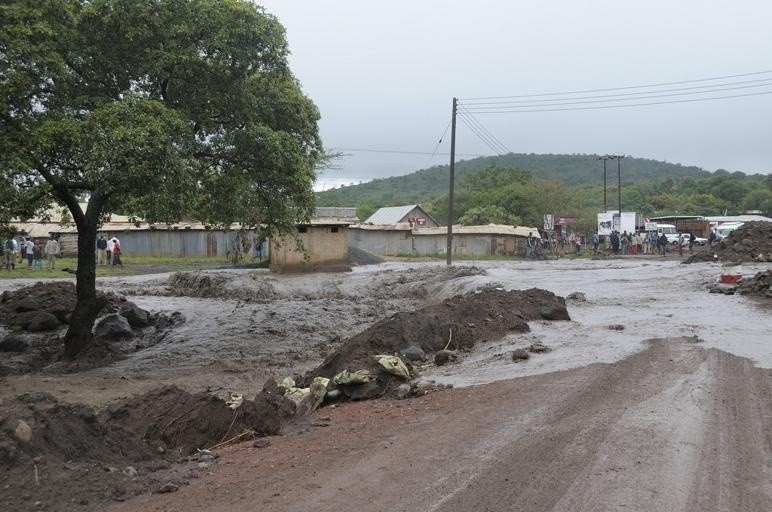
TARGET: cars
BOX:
[667,233,708,246]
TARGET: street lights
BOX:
[598,155,613,213]
[611,155,625,217]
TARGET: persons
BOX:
[1,235,123,273]
[523,227,723,258]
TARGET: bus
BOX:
[657,224,677,241]
[712,222,744,238]
